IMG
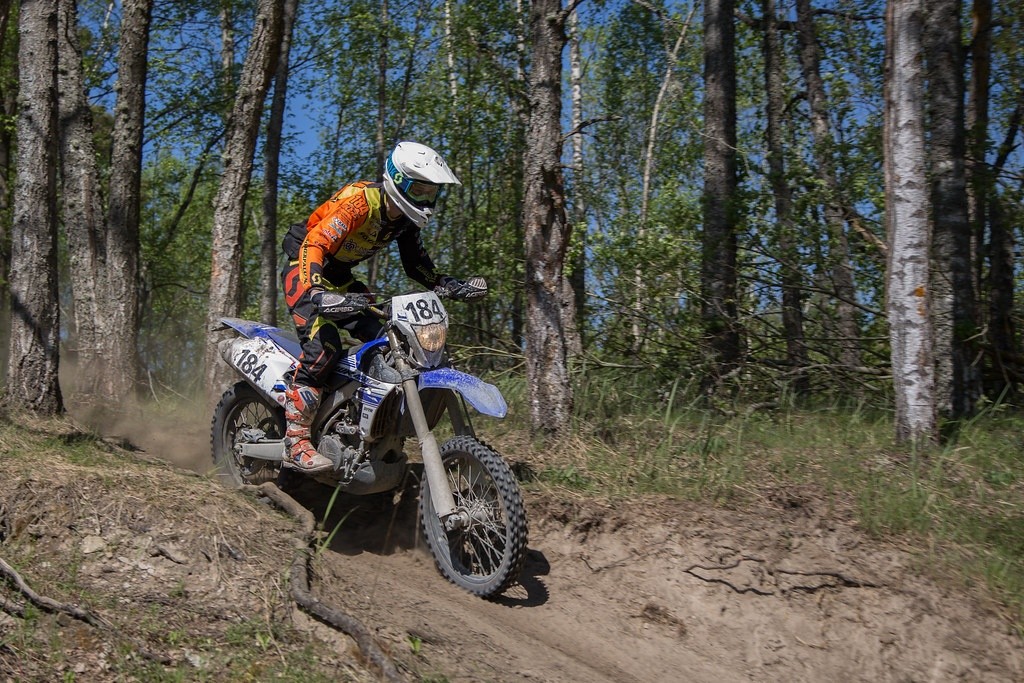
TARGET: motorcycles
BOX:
[208,275,529,602]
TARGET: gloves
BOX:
[435,277,487,304]
[310,292,367,320]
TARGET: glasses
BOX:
[405,182,439,203]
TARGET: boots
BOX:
[281,382,334,473]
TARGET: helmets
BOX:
[383,141,461,227]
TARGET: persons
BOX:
[281,141,487,472]
